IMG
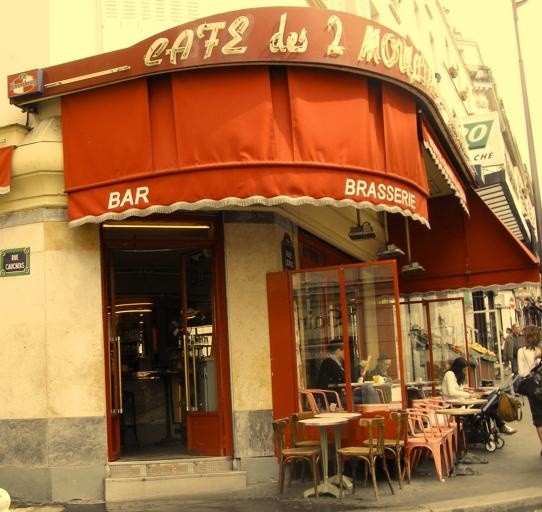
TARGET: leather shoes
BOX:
[499,424,518,435]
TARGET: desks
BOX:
[146,370,184,447]
[435,398,489,477]
[391,380,441,399]
[327,381,387,404]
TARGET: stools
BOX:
[121,391,140,447]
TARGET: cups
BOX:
[373,375,387,385]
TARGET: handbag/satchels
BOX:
[495,391,523,422]
[512,364,542,398]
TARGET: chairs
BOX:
[270,396,468,502]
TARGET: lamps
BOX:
[348,208,426,276]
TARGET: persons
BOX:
[441,357,518,435]
[497,322,542,375]
[318,336,368,404]
[516,324,542,455]
[365,355,393,380]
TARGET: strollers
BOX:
[463,373,523,452]
